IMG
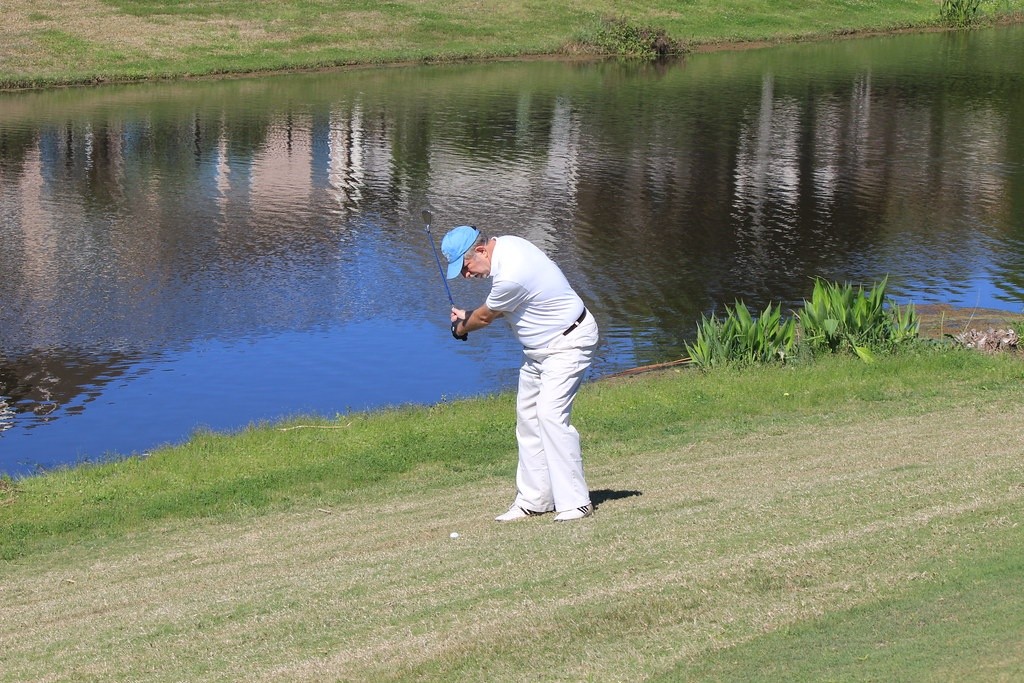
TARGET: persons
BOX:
[441,226,599,520]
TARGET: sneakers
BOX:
[495,503,543,521]
[554,504,593,521]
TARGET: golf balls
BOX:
[450,532,459,540]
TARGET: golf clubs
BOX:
[421,209,468,342]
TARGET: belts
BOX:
[562,307,587,336]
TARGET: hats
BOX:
[441,225,480,280]
